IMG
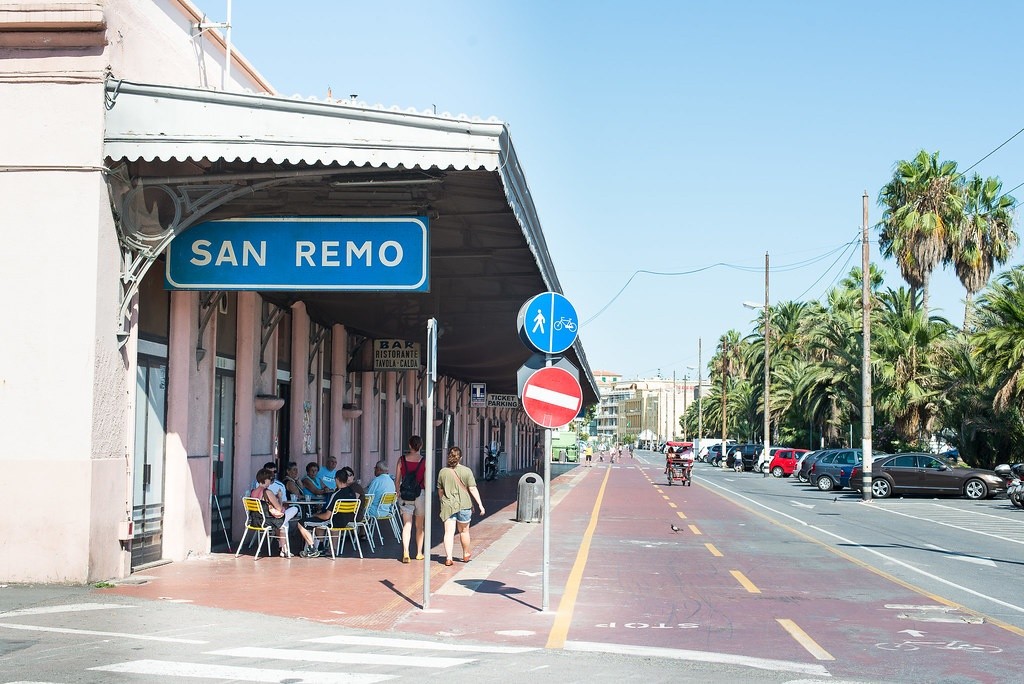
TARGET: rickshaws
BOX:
[664,440,694,486]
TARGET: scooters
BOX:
[994,463,1024,509]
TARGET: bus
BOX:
[552,431,578,462]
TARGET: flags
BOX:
[627,421,630,428]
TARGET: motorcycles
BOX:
[482,441,501,482]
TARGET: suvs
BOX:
[697,444,764,471]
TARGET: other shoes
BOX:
[445,559,453,565]
[463,554,471,562]
[403,553,411,563]
[416,554,425,560]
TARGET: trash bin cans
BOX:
[516,472,544,523]
[559,450,566,463]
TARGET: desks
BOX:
[283,501,327,556]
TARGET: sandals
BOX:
[358,534,366,541]
[279,551,294,557]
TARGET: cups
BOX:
[305,494,312,502]
[290,494,298,501]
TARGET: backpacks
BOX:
[400,455,424,501]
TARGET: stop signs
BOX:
[522,366,583,428]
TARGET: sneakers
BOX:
[299,547,320,557]
[319,547,331,557]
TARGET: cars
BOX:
[770,449,810,478]
[642,443,693,454]
[792,449,894,491]
[758,447,791,474]
[849,453,1008,500]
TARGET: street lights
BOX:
[743,301,769,477]
[687,366,702,439]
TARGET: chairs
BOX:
[234,492,403,561]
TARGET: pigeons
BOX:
[670,524,683,534]
[834,497,838,502]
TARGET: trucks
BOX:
[693,439,737,460]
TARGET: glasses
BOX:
[347,473,354,476]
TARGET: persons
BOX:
[251,456,396,557]
[666,447,693,479]
[533,441,543,474]
[437,447,485,566]
[733,448,742,473]
[585,444,634,466]
[395,436,426,561]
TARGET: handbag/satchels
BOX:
[262,487,285,518]
